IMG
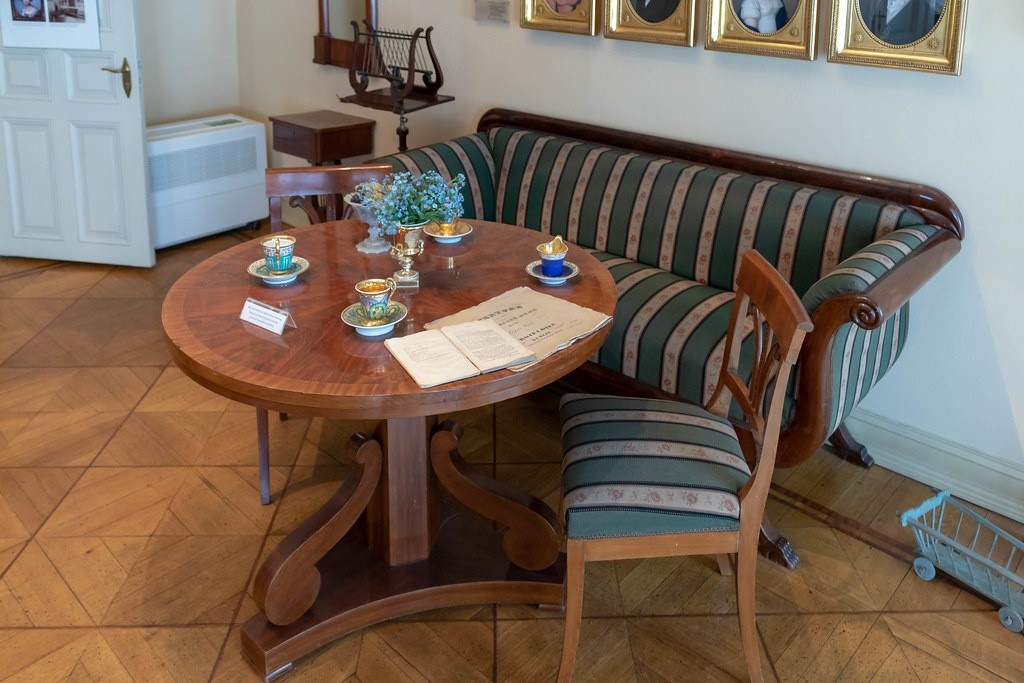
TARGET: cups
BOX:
[536,235,568,277]
[260,234,297,276]
[354,277,397,326]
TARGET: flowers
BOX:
[353,169,466,236]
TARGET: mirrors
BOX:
[312,0,379,75]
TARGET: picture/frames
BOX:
[703,0,820,61]
[604,0,700,48]
[519,0,602,36]
[826,0,969,76]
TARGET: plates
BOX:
[341,301,407,337]
[423,222,474,244]
[246,256,309,285]
[525,259,580,285]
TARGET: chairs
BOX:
[555,249,813,683]
[256,164,394,507]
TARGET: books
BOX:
[384,320,537,390]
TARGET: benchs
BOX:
[361,108,965,570]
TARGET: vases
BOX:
[390,220,430,288]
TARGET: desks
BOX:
[269,109,376,225]
[162,218,618,683]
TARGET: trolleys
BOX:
[897,488,1024,633]
